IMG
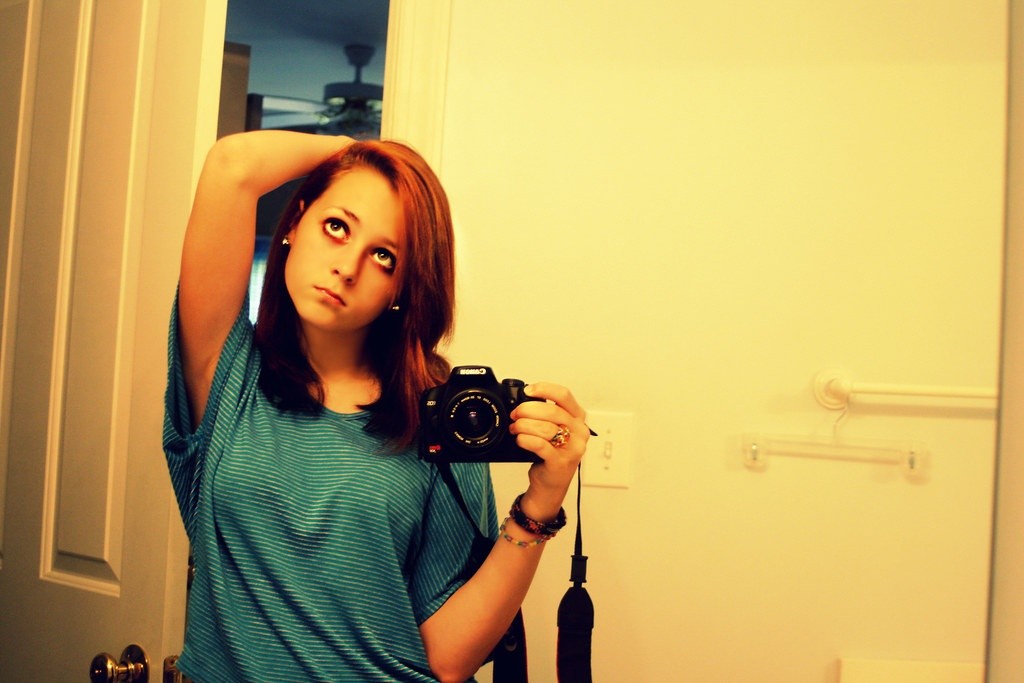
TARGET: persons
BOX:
[159,127,589,683]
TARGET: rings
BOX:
[548,423,571,448]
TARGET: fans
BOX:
[261,42,383,138]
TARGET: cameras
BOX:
[419,362,550,463]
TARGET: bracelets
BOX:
[498,516,557,549]
[509,491,568,535]
